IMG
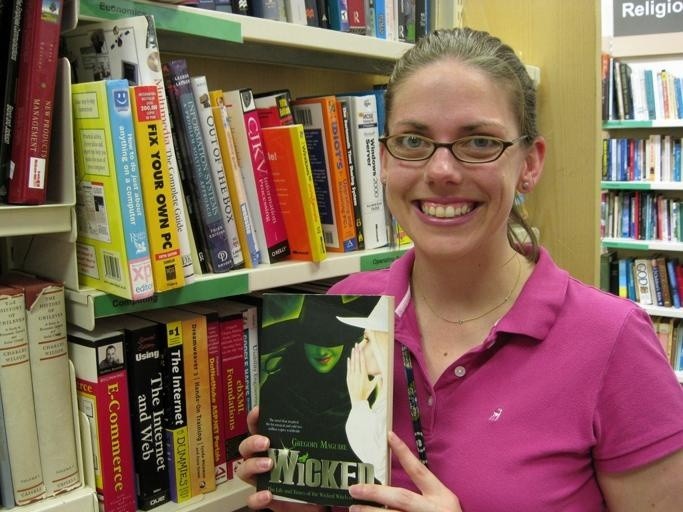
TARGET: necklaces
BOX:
[405,251,524,327]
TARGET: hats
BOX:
[261,295,365,355]
[335,295,388,333]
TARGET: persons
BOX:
[235,23,682,512]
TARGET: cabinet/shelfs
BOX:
[596,51,683,386]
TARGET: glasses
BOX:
[378,133,529,163]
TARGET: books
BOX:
[595,48,682,385]
[0,2,440,512]
